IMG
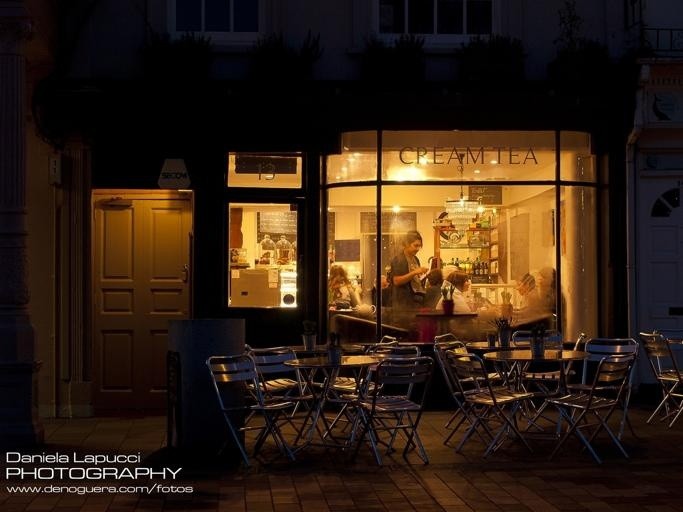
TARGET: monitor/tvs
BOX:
[334,239,361,263]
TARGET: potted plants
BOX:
[442,287,454,313]
[489,314,517,346]
[302,321,316,350]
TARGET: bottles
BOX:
[450,256,488,275]
[275,235,292,265]
[258,234,275,265]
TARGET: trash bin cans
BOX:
[163,318,246,472]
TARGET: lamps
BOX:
[458,153,464,206]
[477,201,484,214]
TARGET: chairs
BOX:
[206,330,682,466]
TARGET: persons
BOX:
[509,272,539,321]
[422,267,440,310]
[432,270,475,311]
[327,262,361,312]
[441,265,461,280]
[536,265,556,316]
[390,231,428,326]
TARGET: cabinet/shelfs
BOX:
[434,221,490,283]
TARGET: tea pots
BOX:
[355,304,376,318]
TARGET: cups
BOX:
[486,332,496,348]
